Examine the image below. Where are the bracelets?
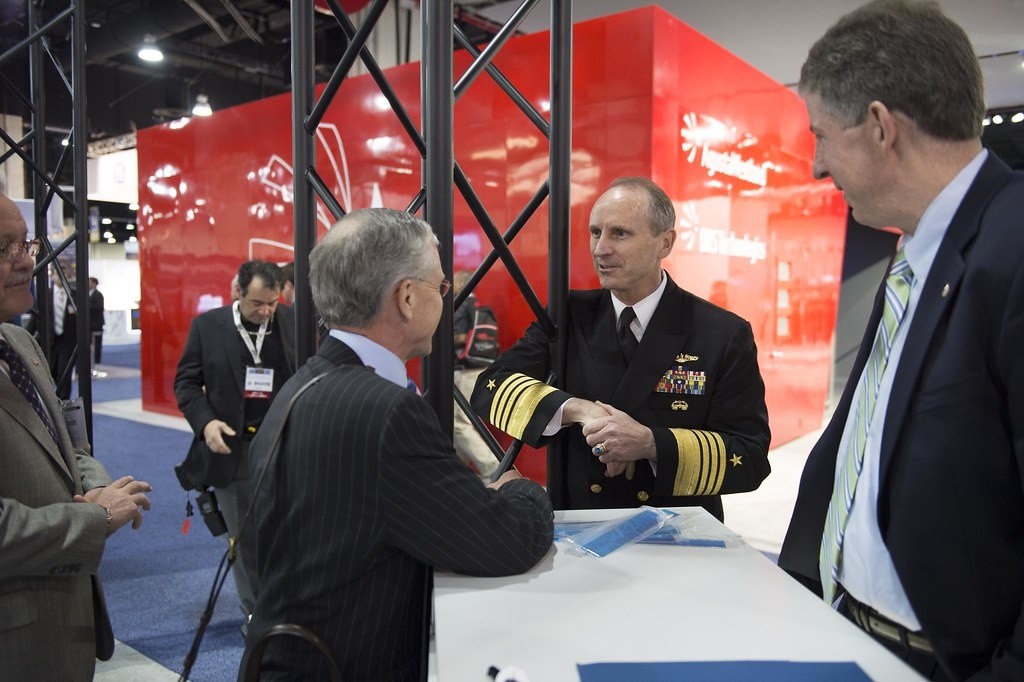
[100,504,112,527]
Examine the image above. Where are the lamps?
[191,93,213,118]
[138,32,164,63]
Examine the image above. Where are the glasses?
[394,279,451,297]
[0,239,41,260]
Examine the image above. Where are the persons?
[89,278,105,363]
[236,207,555,682]
[23,265,76,399]
[778,0,1024,682]
[468,177,772,524]
[174,260,295,621]
[453,270,500,478]
[0,194,152,682]
[280,262,296,309]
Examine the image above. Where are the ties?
[616,307,639,368]
[406,377,422,398]
[0,340,64,460]
[55,291,64,336]
[818,245,915,607]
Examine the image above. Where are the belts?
[845,601,933,654]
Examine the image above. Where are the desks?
[428,506,929,681]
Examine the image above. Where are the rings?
[595,443,607,454]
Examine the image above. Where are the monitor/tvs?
[88,200,139,244]
